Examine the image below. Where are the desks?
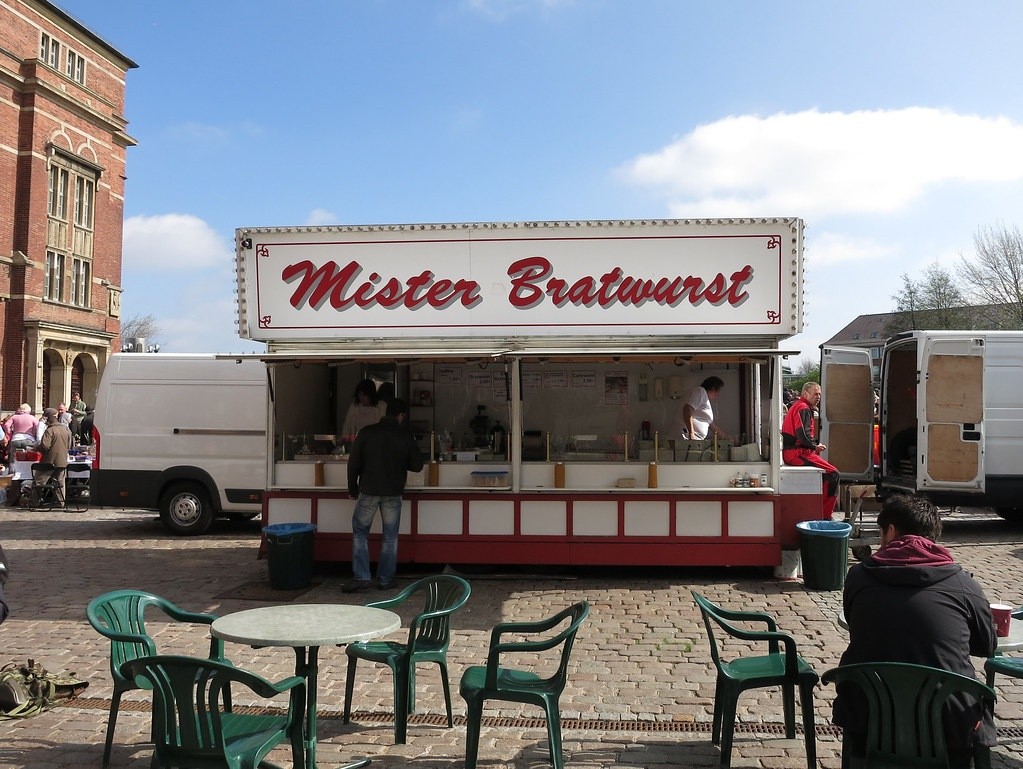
[210,603,402,769]
[838,608,1023,653]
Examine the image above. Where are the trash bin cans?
[795,520,853,592]
[262,523,318,593]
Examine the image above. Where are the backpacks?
[19,480,39,506]
[0,657,89,718]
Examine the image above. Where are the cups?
[990,604,1012,636]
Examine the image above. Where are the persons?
[0,423,8,447]
[24,416,72,510]
[81,405,95,447]
[68,392,87,426]
[339,398,424,596]
[5,403,39,468]
[58,404,72,429]
[339,380,387,452]
[833,494,999,768]
[783,381,841,520]
[376,382,394,401]
[784,386,801,417]
[39,408,59,451]
[667,376,737,448]
[69,414,82,448]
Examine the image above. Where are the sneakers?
[341,579,370,592]
[378,579,398,590]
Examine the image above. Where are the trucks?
[86,353,267,534]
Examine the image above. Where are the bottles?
[736,471,750,488]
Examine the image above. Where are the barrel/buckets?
[490,420,504,454]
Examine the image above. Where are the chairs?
[63,463,104,513]
[344,574,472,745]
[119,654,306,769]
[85,589,225,769]
[28,462,68,512]
[820,660,999,769]
[690,589,821,769]
[457,599,591,769]
[983,657,1023,720]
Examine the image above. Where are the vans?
[818,330,1022,528]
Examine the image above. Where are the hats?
[44,407,58,415]
[85,405,94,410]
